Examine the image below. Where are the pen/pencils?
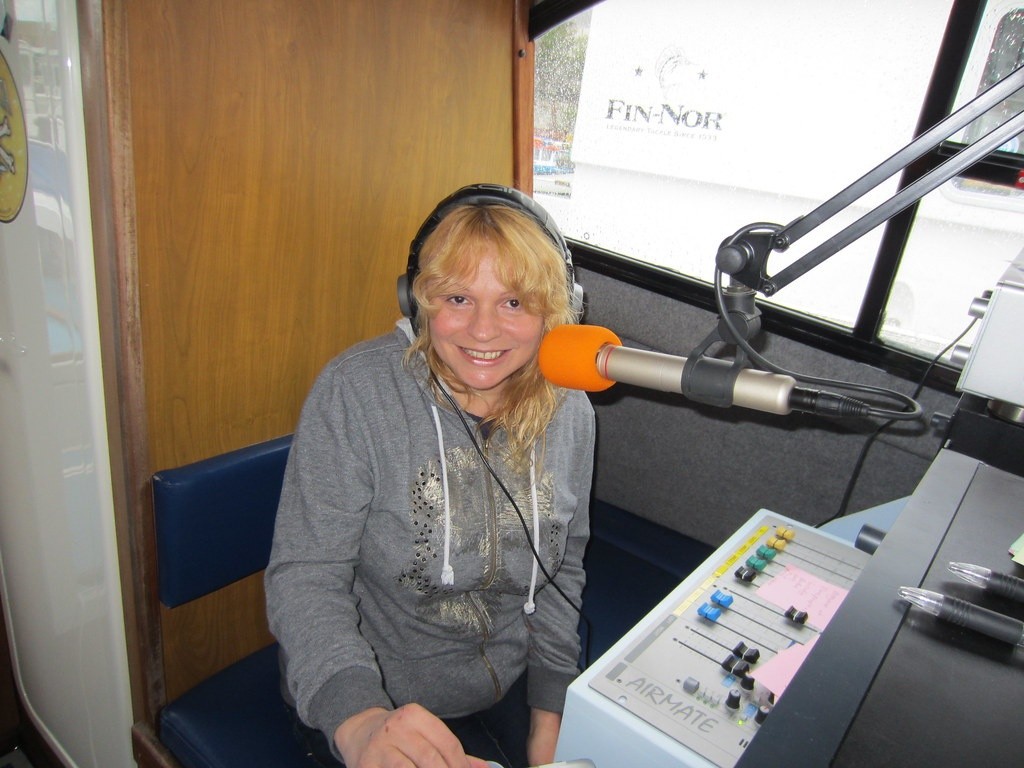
[946,560,1024,603]
[899,586,1024,649]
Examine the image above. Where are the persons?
[263,184,598,768]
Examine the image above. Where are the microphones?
[538,324,796,415]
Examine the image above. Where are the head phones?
[397,183,583,337]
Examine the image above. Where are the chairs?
[133,429,722,768]
